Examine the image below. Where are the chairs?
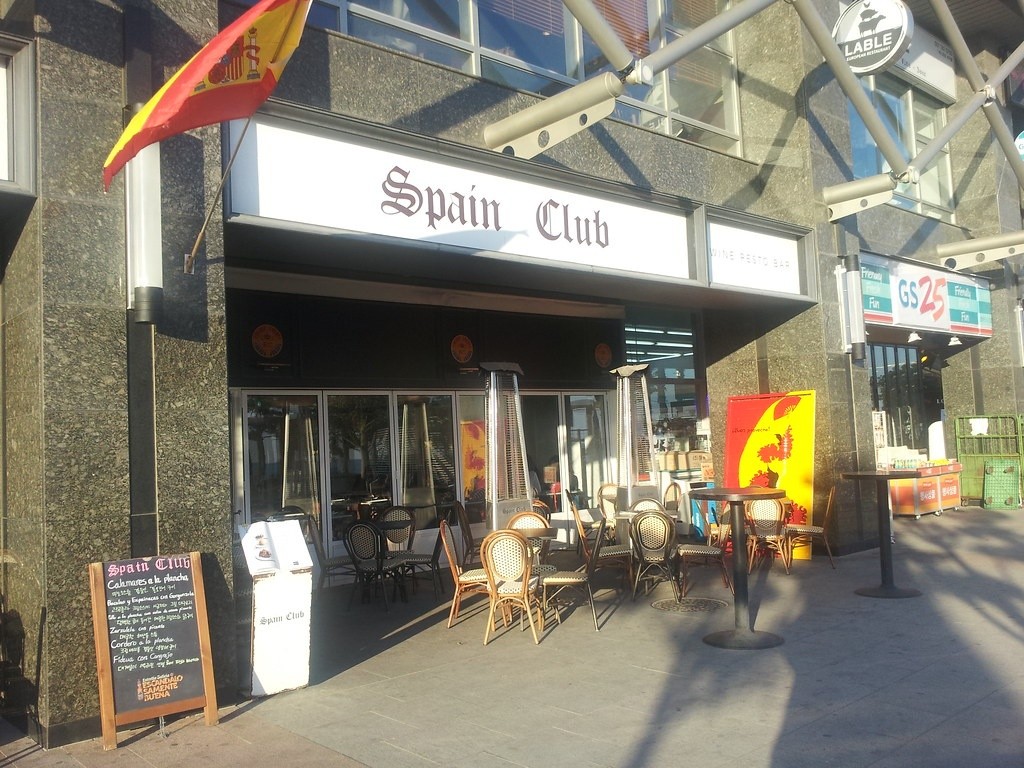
[308,483,837,646]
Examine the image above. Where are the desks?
[614,509,678,578]
[840,471,923,598]
[688,487,785,650]
[335,519,415,603]
[598,493,616,501]
[513,527,557,538]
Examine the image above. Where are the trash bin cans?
[486,498,532,535]
[615,484,661,547]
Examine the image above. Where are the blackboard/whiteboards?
[89,549,216,727]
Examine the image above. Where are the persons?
[527,454,555,512]
[548,456,580,510]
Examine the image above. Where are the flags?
[103,0,310,188]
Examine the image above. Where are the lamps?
[907,332,922,343]
[121,101,163,322]
[948,335,962,346]
[836,254,866,361]
